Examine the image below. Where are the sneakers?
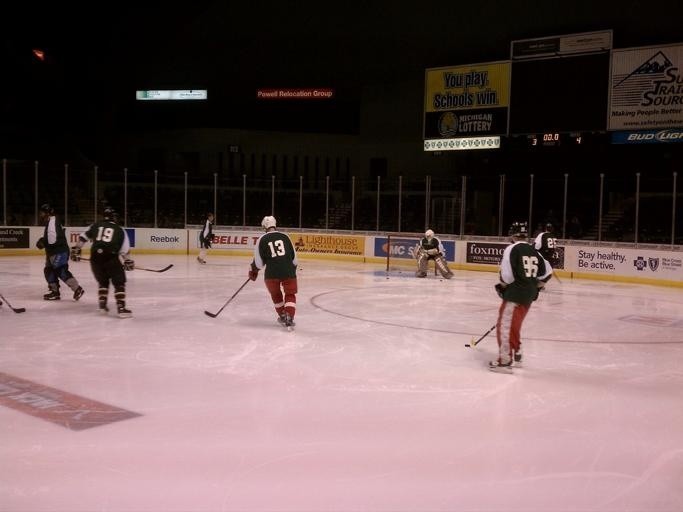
[277,314,296,327]
[118,306,132,315]
[43,292,61,298]
[487,360,513,369]
[73,286,83,299]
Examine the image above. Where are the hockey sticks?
[463,324,497,347]
[0,294,25,314]
[204,278,249,318]
[79,257,174,274]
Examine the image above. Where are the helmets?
[103,206,120,222]
[40,203,55,215]
[261,215,277,232]
[509,222,529,240]
[425,230,435,240]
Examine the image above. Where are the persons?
[533,223,558,291]
[248,216,297,332]
[414,229,456,280]
[36,203,85,302]
[487,218,554,375]
[196,212,214,265]
[71,206,135,319]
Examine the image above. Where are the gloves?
[70,246,81,261]
[123,260,135,271]
